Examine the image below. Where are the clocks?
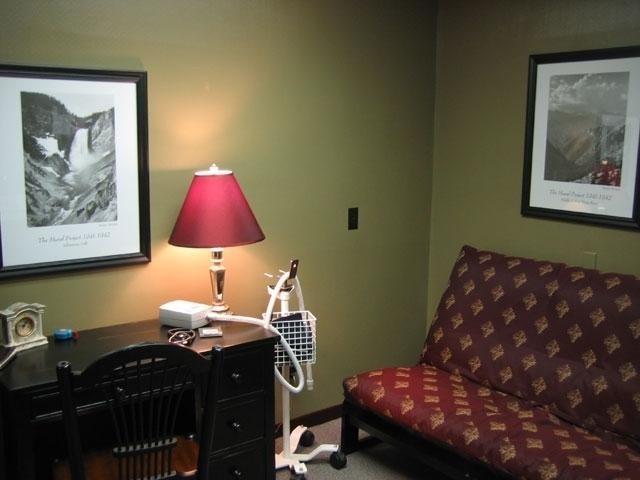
[0,302,49,352]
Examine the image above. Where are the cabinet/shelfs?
[1,310,281,479]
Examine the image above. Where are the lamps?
[168,163,264,319]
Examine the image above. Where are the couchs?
[339,247,640,480]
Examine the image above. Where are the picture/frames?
[519,47,640,234]
[0,63,150,282]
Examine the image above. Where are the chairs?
[54,341,221,480]
